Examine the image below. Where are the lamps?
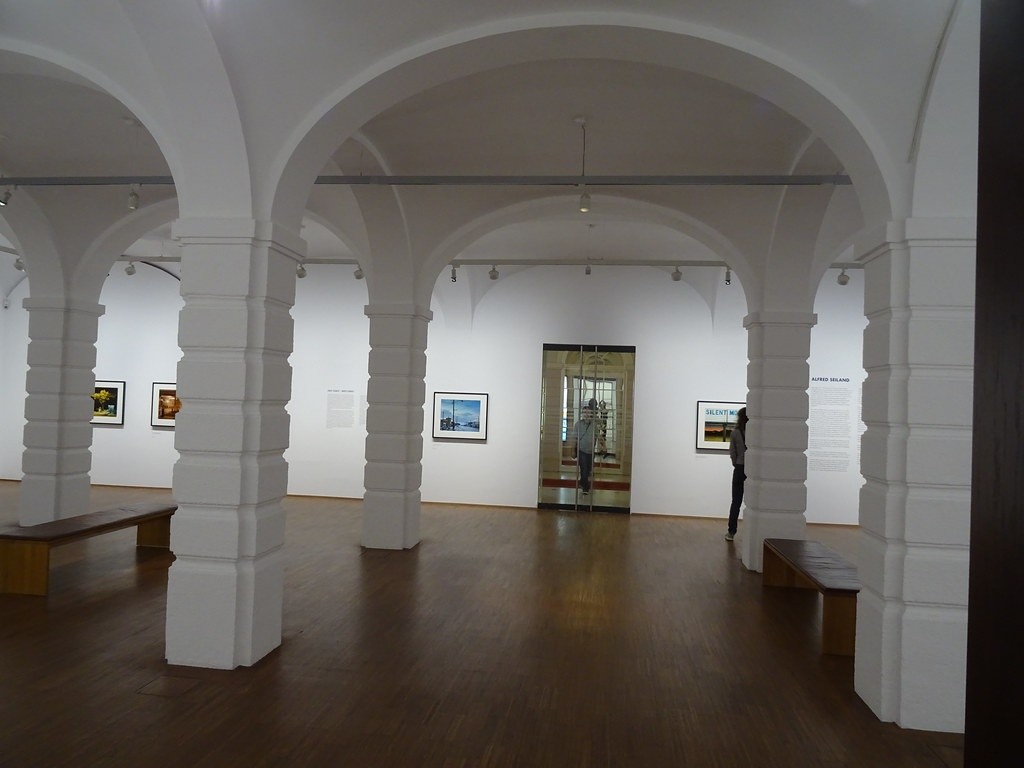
[838,268,849,284]
[354,264,364,280]
[725,267,732,285]
[13,259,26,272]
[124,262,136,274]
[296,263,306,278]
[128,192,138,209]
[488,265,499,279]
[0,188,12,206]
[451,265,457,283]
[671,267,681,281]
[574,116,592,212]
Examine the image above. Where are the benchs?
[759,537,863,661]
[0,501,179,599]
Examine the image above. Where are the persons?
[588,398,608,459]
[571,406,598,495]
[725,407,749,540]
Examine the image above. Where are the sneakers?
[724,533,733,540]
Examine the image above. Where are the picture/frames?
[432,391,488,440]
[93,380,126,425]
[696,400,745,453]
[151,381,177,428]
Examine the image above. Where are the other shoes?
[582,489,589,495]
[602,435,606,437]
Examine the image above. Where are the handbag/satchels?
[572,441,578,458]
